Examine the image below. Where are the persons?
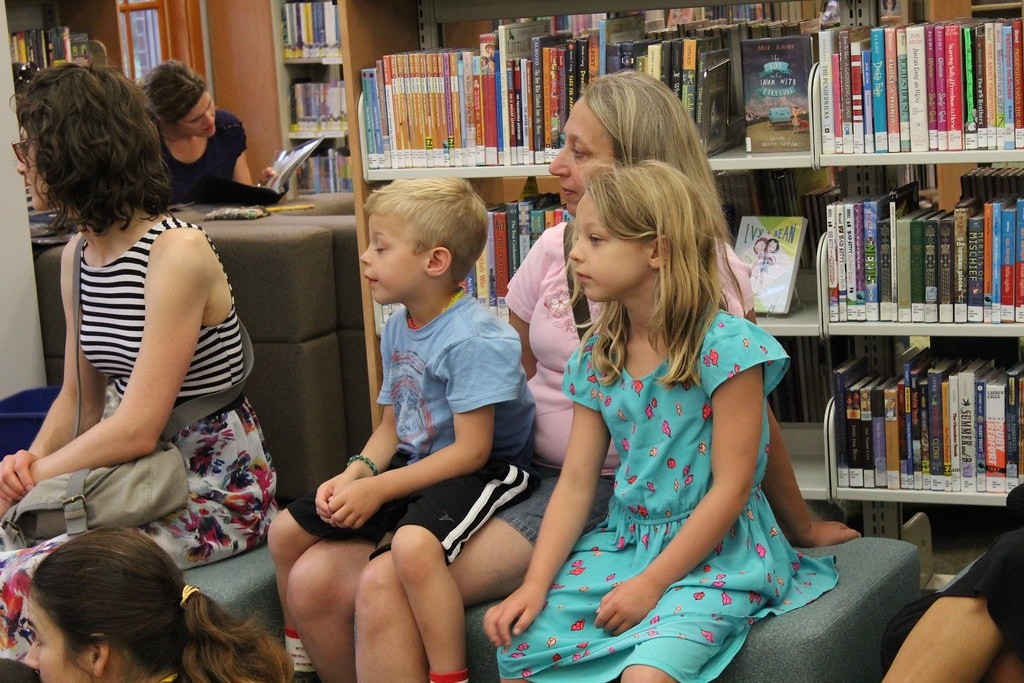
[269,72,860,683]
[0,58,294,683]
[882,484,1024,683]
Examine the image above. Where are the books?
[766,335,1024,493]
[283,0,353,195]
[365,3,1024,168]
[185,135,326,206]
[9,26,89,69]
[370,162,1024,325]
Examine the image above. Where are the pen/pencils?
[267,203,316,209]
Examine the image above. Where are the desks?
[34,190,372,497]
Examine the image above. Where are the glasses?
[11,137,38,163]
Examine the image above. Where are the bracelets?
[347,455,378,476]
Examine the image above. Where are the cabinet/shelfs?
[332,0,1024,594]
[269,0,354,195]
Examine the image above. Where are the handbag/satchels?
[2,440,189,548]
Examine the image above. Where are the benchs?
[462,538,920,683]
[183,525,282,651]
